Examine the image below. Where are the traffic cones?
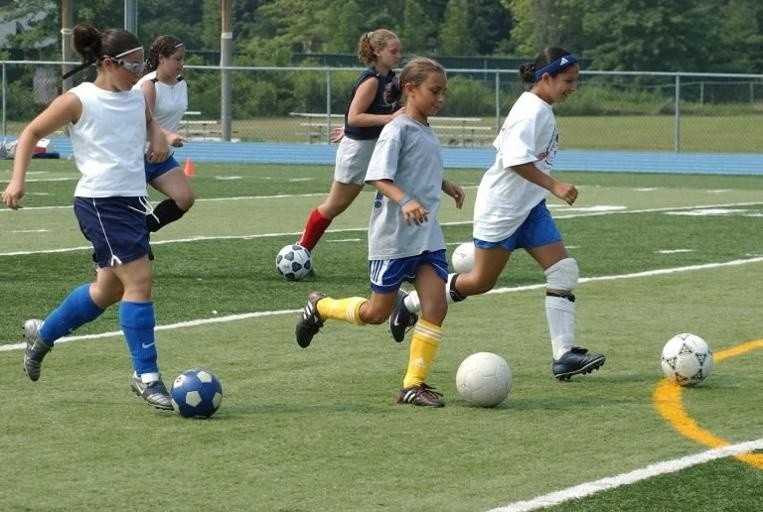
[183,158,195,176]
[184,158,193,176]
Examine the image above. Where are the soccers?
[660,332,714,387]
[455,351,513,408]
[451,242,476,275]
[276,244,313,281]
[171,367,224,421]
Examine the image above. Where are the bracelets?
[398,193,415,207]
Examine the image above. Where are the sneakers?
[295,291,327,348]
[551,346,606,381]
[22,317,54,382]
[387,288,417,342]
[129,370,173,410]
[395,382,445,407]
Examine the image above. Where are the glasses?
[102,53,146,73]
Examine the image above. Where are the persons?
[295,57,465,408]
[380,45,606,381]
[1,22,175,412]
[292,28,410,277]
[130,35,195,233]
